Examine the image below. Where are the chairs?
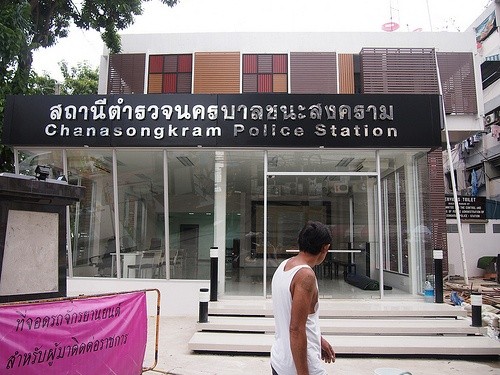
[127,249,185,279]
[89,237,115,277]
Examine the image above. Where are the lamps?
[34,165,50,180]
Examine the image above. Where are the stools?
[315,259,356,280]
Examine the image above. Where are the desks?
[110,249,141,279]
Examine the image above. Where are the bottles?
[424,281,434,303]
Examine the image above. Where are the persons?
[270,220,336,375]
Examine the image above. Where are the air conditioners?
[484,112,495,126]
